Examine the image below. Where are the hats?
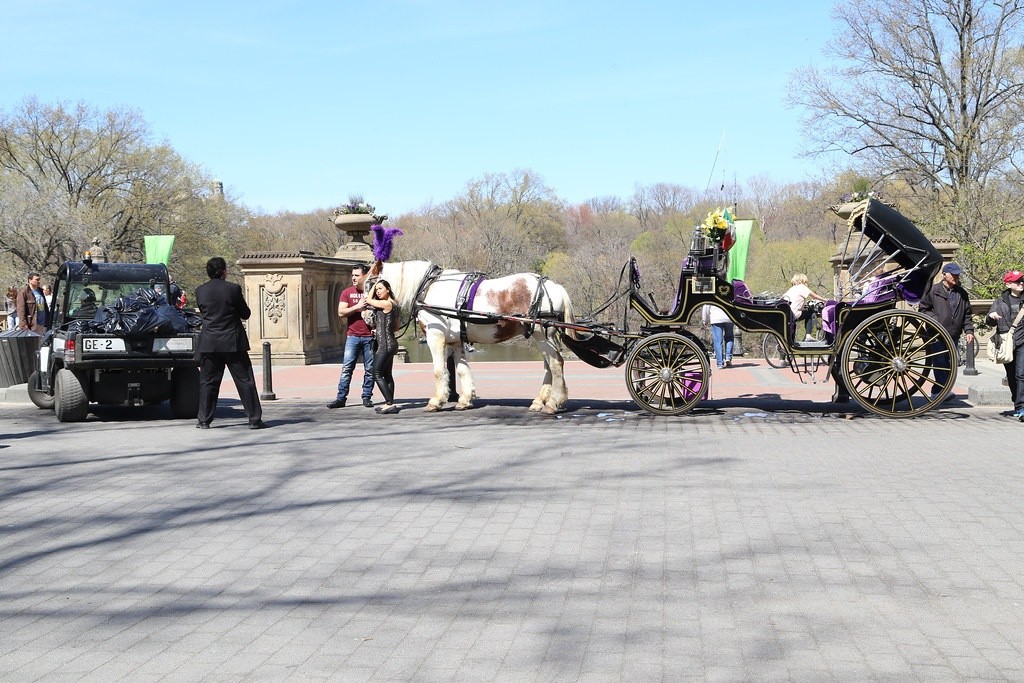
[1003,271,1024,283]
[942,263,965,274]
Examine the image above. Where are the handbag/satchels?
[986,332,1013,364]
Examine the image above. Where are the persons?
[195,257,264,429]
[327,264,375,407]
[917,263,974,402]
[4,286,20,330]
[16,273,50,340]
[702,305,734,368]
[366,279,400,414]
[73,288,97,315]
[43,284,58,313]
[986,271,1024,416]
[154,275,181,307]
[782,273,827,342]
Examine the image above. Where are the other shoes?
[718,366,723,369]
[931,390,955,402]
[375,404,398,414]
[779,361,791,367]
[725,360,732,367]
[805,337,817,342]
[326,400,345,408]
[1014,408,1024,421]
[363,399,373,407]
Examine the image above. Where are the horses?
[361,259,577,414]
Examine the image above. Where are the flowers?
[700,203,738,246]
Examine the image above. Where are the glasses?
[1015,277,1024,284]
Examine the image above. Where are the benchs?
[732,279,796,345]
[822,270,905,344]
[668,256,722,323]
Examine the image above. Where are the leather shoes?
[250,422,269,428]
[196,421,209,429]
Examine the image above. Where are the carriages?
[365,188,962,421]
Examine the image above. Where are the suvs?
[26,249,203,422]
